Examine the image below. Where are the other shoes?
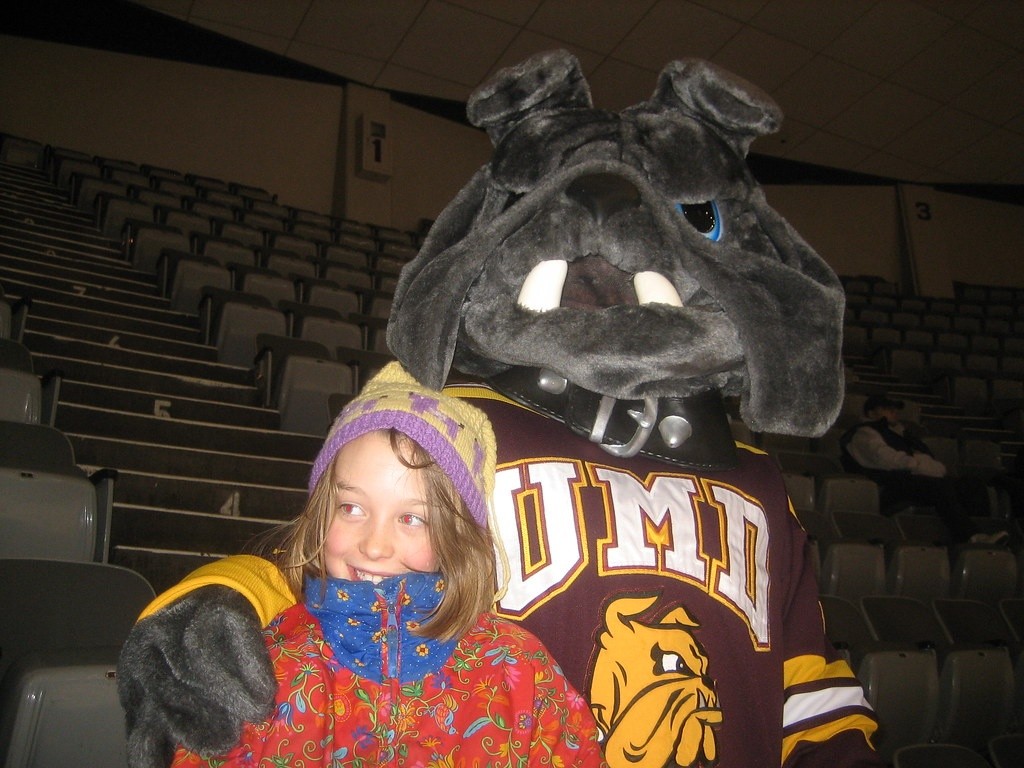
[969,531,1009,547]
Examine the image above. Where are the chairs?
[0,134,1024,768]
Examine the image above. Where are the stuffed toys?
[116,48,879,768]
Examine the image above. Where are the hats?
[863,394,904,413]
[310,362,495,531]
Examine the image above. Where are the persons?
[839,389,1009,547]
[168,361,608,768]
